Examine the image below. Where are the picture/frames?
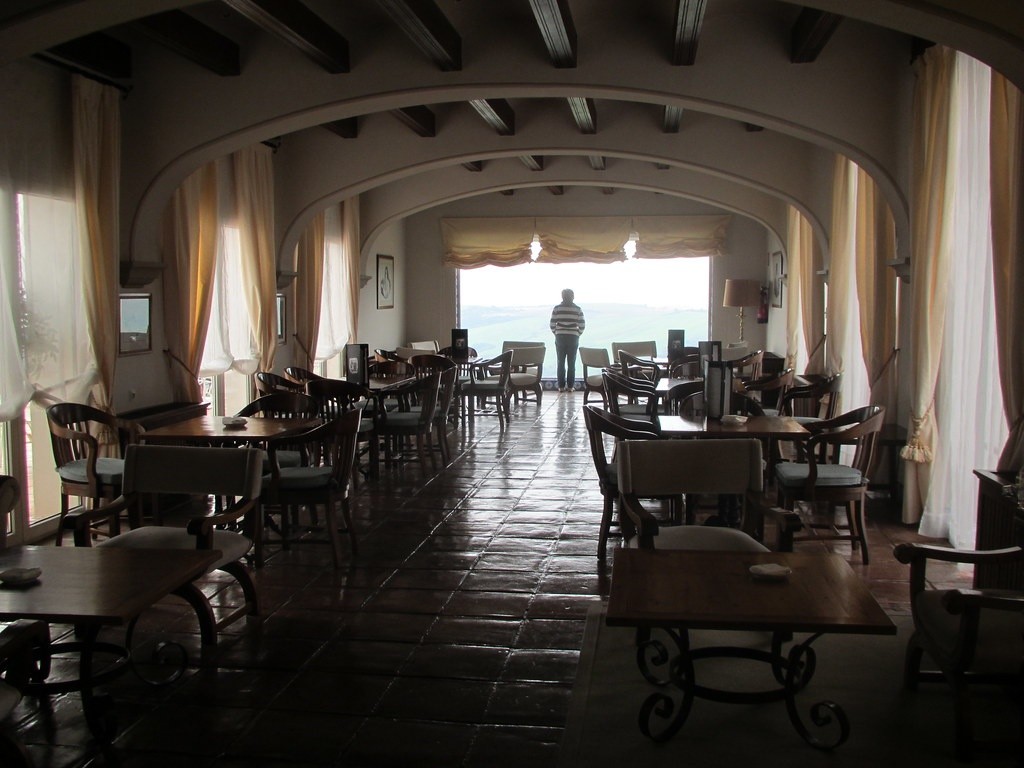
[770,251,782,308]
[376,255,394,310]
[118,293,153,357]
[276,293,287,345]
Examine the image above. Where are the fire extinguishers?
[757,286,769,320]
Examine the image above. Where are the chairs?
[0,329,1024,768]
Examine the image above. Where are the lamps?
[722,278,761,343]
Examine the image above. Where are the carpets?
[554,600,1024,768]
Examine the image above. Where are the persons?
[550,288,585,392]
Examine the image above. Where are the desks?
[607,548,897,751]
[0,543,223,749]
[655,378,746,397]
[334,376,417,481]
[447,357,483,364]
[830,423,907,504]
[656,415,811,527]
[138,416,322,560]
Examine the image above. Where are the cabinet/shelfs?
[792,374,828,417]
[118,402,210,519]
[751,350,784,404]
[972,469,1024,592]
